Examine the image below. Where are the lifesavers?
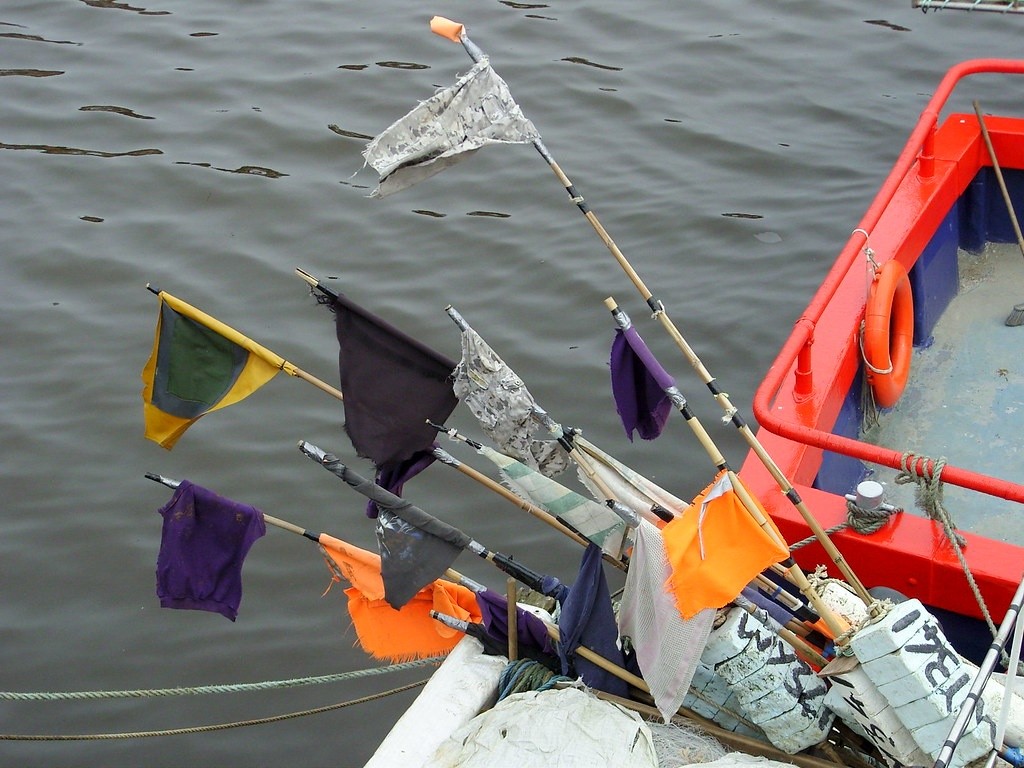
[863,260,914,407]
